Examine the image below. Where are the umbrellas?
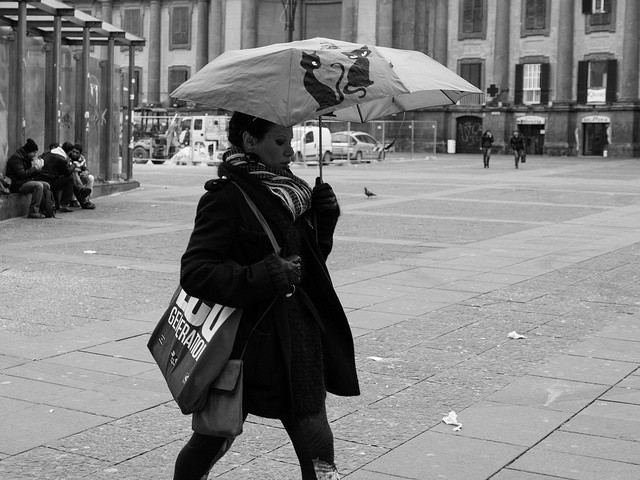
[170,37,485,181]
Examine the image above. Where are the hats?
[23,138,38,153]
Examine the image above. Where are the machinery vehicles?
[119,108,171,164]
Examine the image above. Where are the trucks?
[170,113,232,166]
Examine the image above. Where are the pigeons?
[365,187,376,198]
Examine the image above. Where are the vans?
[291,126,332,165]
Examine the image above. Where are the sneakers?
[27,212,45,219]
[76,188,91,203]
[73,200,80,207]
[58,204,73,212]
[82,201,95,209]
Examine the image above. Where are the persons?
[509,131,525,168]
[184,125,190,145]
[69,144,94,207]
[49,142,91,208]
[41,142,74,212]
[480,130,494,168]
[173,112,361,479]
[6,138,51,218]
[179,125,186,143]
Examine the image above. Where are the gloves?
[313,173,339,222]
[257,249,303,288]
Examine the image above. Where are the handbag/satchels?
[145,285,243,415]
[191,360,243,438]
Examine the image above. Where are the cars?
[331,131,385,162]
